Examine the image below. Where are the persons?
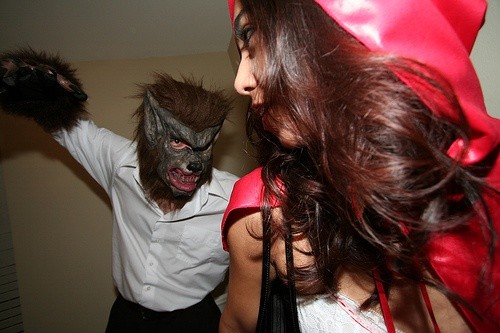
[221,0,500,333]
[0,47,242,333]
